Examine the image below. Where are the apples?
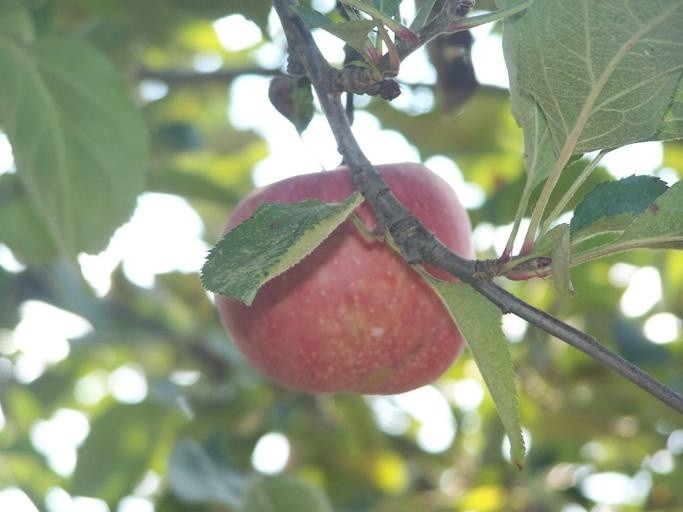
[215,162,474,394]
